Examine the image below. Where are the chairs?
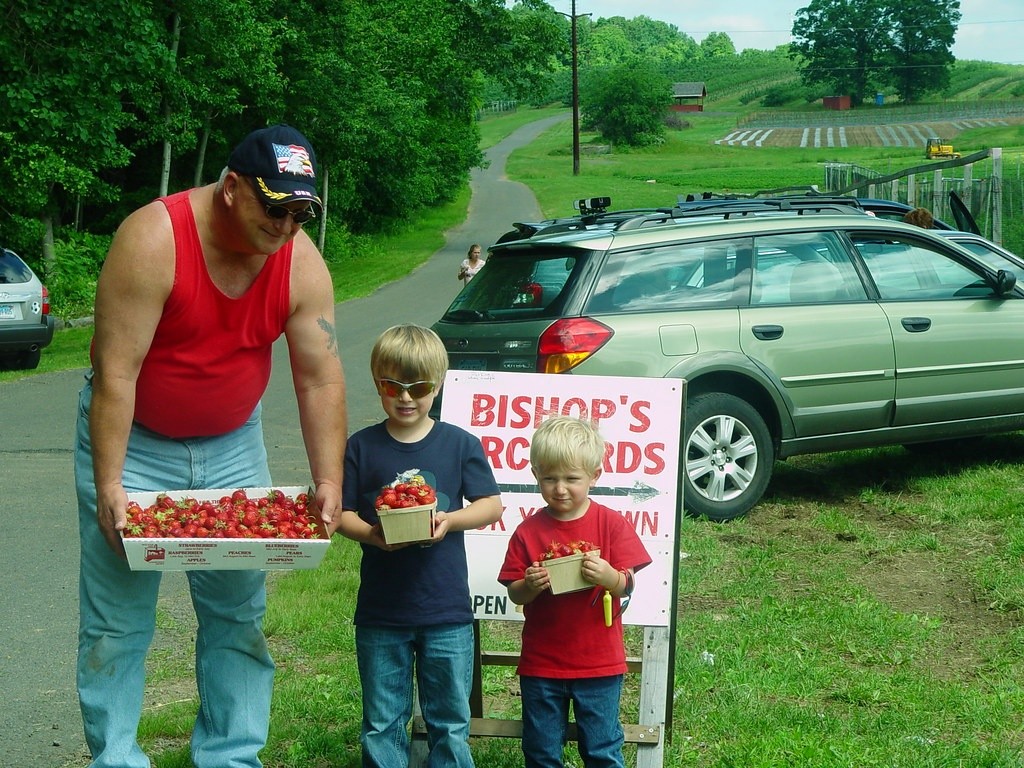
[704,267,763,304]
[789,260,849,302]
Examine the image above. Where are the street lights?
[553,0,593,177]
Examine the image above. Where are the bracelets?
[603,572,621,626]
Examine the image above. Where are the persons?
[497,413,653,768]
[904,207,935,230]
[74,125,348,768]
[335,322,503,768]
[458,244,485,287]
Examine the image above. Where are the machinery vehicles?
[926,136,962,161]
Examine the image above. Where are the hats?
[228,123,323,214]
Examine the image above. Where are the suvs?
[431,190,1024,522]
[1,248,56,371]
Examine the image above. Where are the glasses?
[374,378,436,399]
[244,176,316,224]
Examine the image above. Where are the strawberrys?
[538,539,594,562]
[121,488,321,539]
[375,479,437,511]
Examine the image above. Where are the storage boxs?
[542,550,601,594]
[376,498,437,545]
